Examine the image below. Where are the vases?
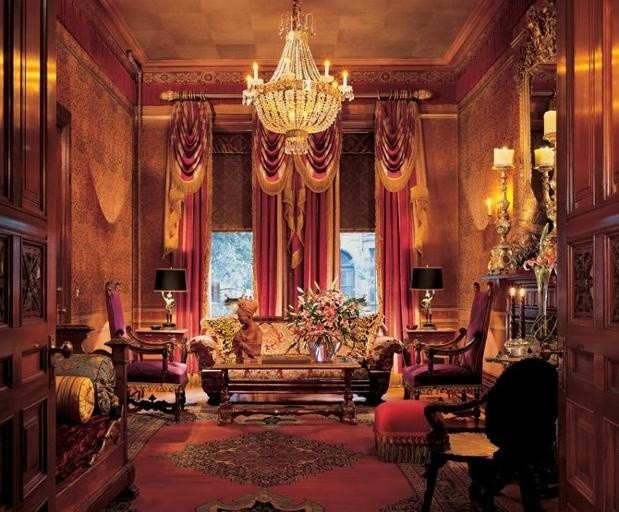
[308,341,340,362]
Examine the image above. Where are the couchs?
[186,311,404,406]
[54,321,139,512]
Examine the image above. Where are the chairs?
[402,281,493,423]
[104,280,189,423]
[422,357,559,512]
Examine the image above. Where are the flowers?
[283,278,367,358]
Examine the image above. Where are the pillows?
[53,352,119,417]
[55,374,95,424]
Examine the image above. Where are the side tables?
[403,328,457,344]
[135,326,187,361]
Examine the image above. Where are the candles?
[507,288,516,340]
[543,110,556,134]
[534,146,554,165]
[493,146,514,164]
[519,289,527,338]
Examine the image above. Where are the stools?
[374,400,429,464]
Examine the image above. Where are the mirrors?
[514,4,557,212]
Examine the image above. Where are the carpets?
[107,400,523,512]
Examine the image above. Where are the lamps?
[410,267,444,328]
[241,0,355,156]
[153,267,187,328]
